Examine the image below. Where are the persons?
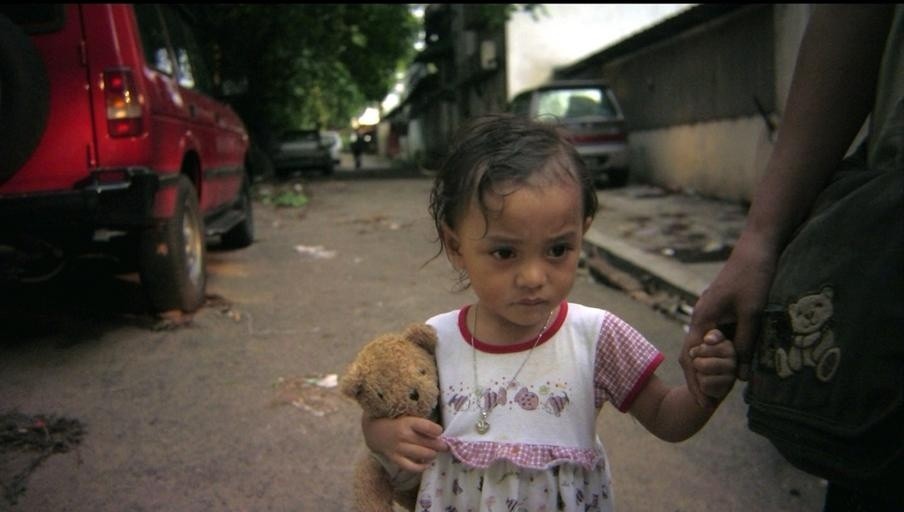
[360,108,738,512]
[678,3,904,512]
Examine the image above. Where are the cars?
[270,125,344,181]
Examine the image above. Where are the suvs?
[0,0,257,317]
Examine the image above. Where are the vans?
[504,76,630,189]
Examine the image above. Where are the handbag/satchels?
[740,147,903,501]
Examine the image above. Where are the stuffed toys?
[337,321,440,512]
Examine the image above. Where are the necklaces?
[472,299,554,434]
